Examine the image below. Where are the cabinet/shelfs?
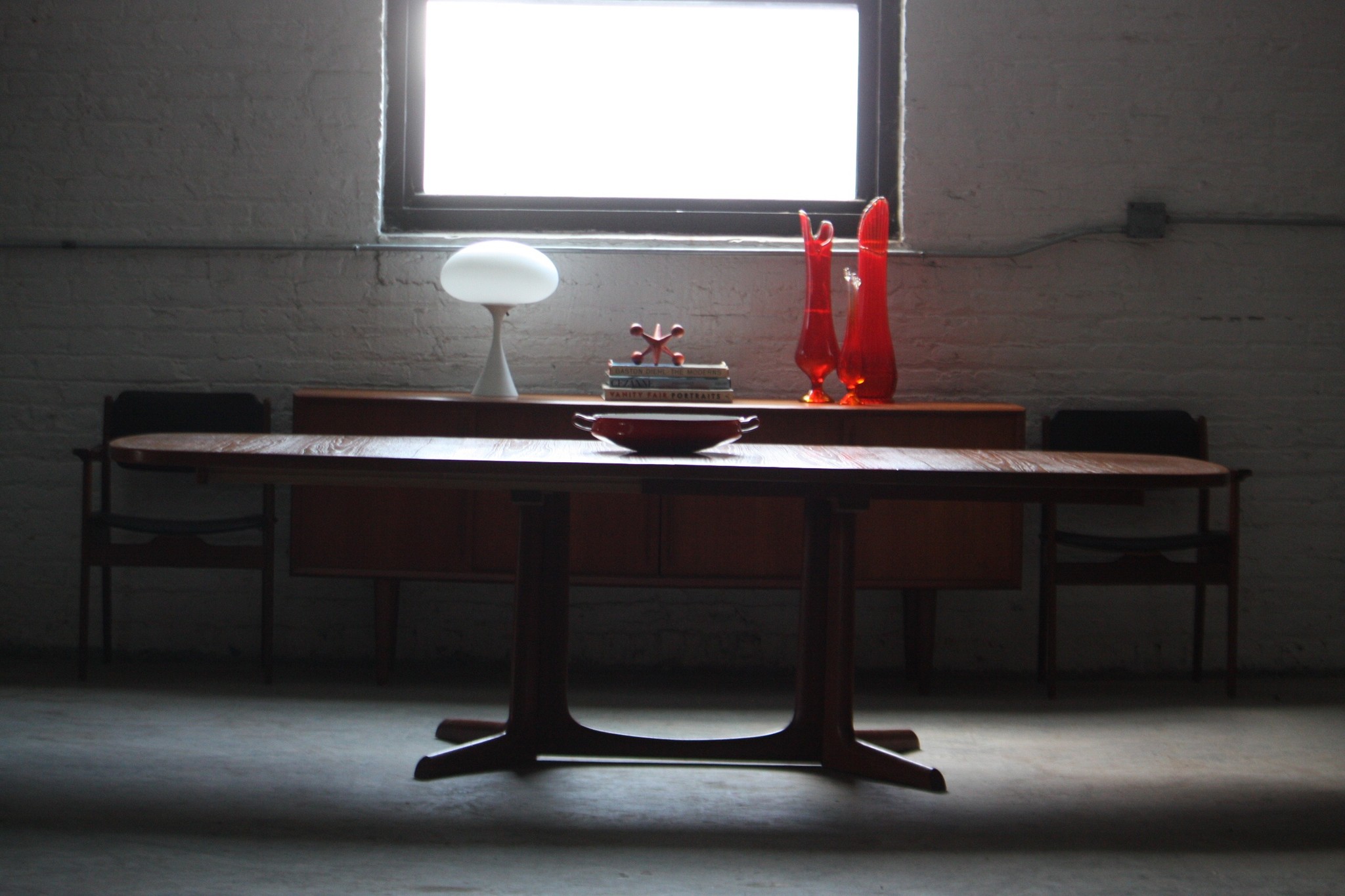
[289,391,1029,690]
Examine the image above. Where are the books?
[602,362,734,403]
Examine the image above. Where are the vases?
[839,196,903,404]
[794,209,839,406]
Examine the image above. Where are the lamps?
[440,241,559,401]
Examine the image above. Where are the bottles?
[838,196,900,406]
[793,209,841,403]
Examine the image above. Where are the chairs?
[1026,409,1242,689]
[75,387,285,675]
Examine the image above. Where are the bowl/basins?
[572,410,761,456]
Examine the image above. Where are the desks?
[111,427,1232,789]
